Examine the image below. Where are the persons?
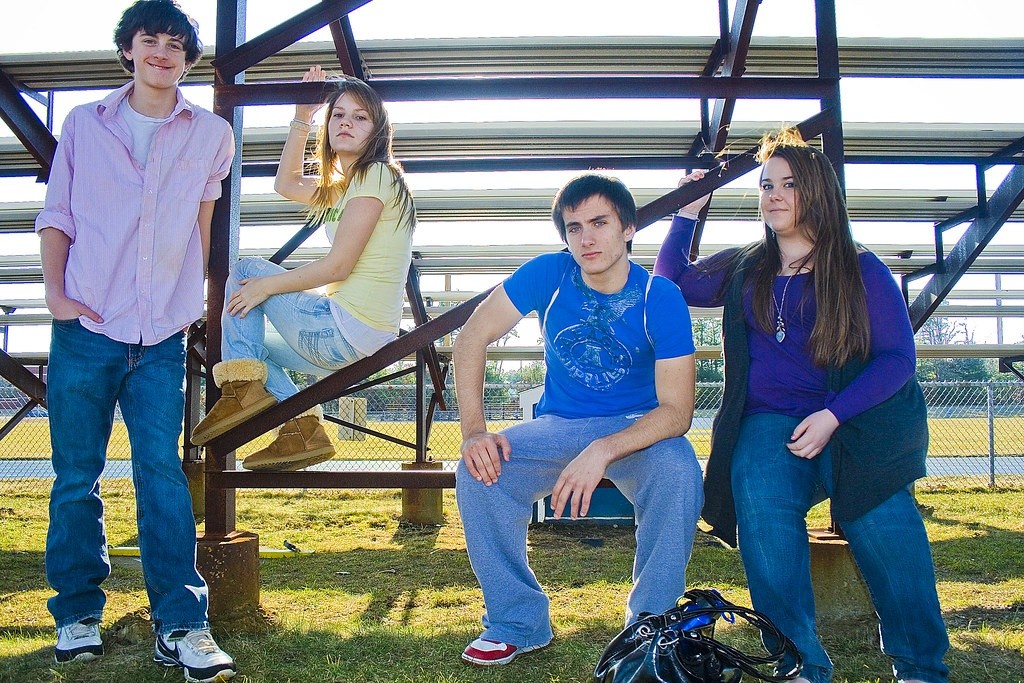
[36,0,238,683]
[653,141,954,683]
[452,173,705,666]
[190,64,419,471]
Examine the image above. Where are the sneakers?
[54,617,104,665]
[461,636,554,666]
[152,627,237,683]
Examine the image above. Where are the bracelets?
[290,119,312,132]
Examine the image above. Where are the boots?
[242,403,337,471]
[190,359,277,447]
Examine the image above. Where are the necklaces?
[772,269,799,343]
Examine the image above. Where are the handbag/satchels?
[593,588,805,683]
